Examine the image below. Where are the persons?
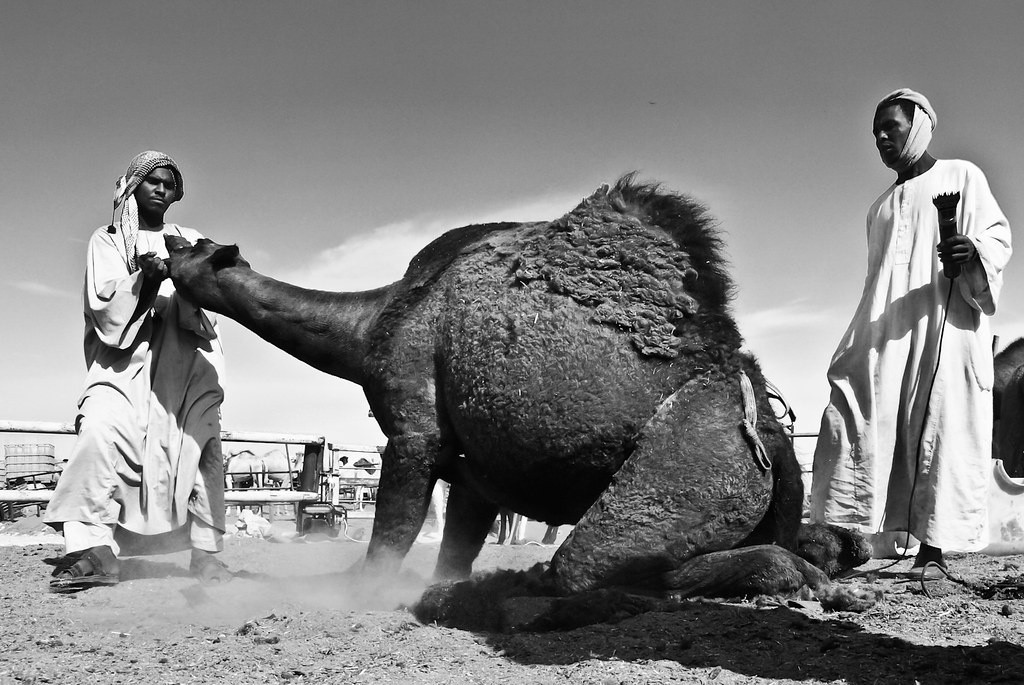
[810,88,1023,560]
[42,149,242,595]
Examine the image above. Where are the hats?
[108,150,184,235]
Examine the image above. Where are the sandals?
[50,551,127,588]
[188,553,230,588]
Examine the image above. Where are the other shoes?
[908,549,944,579]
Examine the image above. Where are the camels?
[162,173,875,634]
